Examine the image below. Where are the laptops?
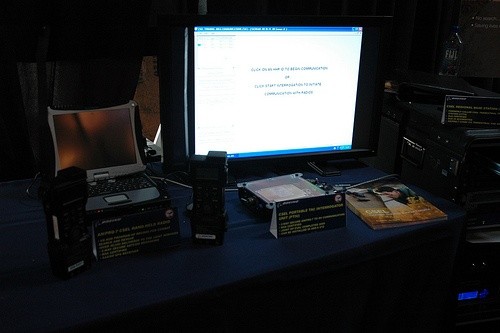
[47,99,160,212]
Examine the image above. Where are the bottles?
[438,25,464,77]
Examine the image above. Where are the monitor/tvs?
[185,14,394,185]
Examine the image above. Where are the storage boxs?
[236,172,329,223]
[90,189,182,261]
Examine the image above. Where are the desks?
[0,163,466,333]
